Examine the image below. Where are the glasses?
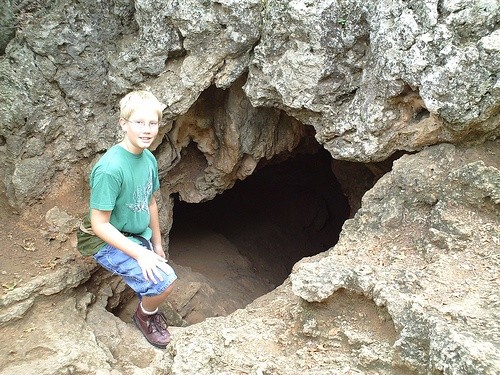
[123,116,161,128]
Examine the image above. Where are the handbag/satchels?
[76,212,152,258]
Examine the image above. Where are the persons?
[90,91,177,348]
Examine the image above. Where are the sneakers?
[132,301,172,347]
